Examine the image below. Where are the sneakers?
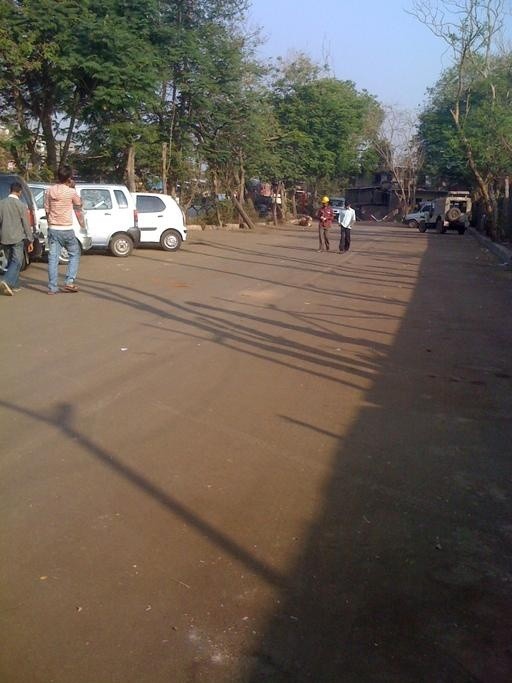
[64,284,80,292]
[0,280,21,296]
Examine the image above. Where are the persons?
[317,196,356,254]
[0,182,35,296]
[43,165,81,295]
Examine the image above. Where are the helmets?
[321,196,330,203]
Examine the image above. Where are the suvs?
[399,201,435,231]
[416,190,474,237]
[327,195,347,220]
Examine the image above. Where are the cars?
[0,183,187,274]
